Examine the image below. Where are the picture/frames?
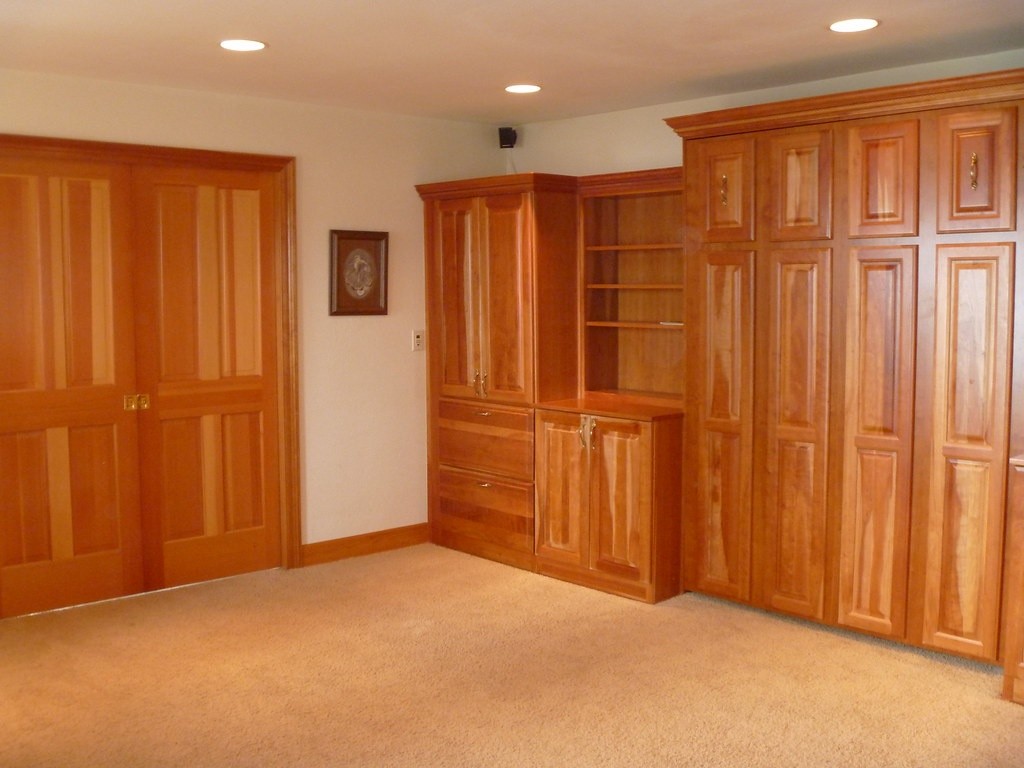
[327,229,390,317]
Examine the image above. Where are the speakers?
[499,127,514,149]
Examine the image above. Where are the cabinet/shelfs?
[578,165,684,405]
[429,463,534,575]
[413,173,578,404]
[532,397,683,605]
[660,66,1023,708]
[427,395,537,483]
